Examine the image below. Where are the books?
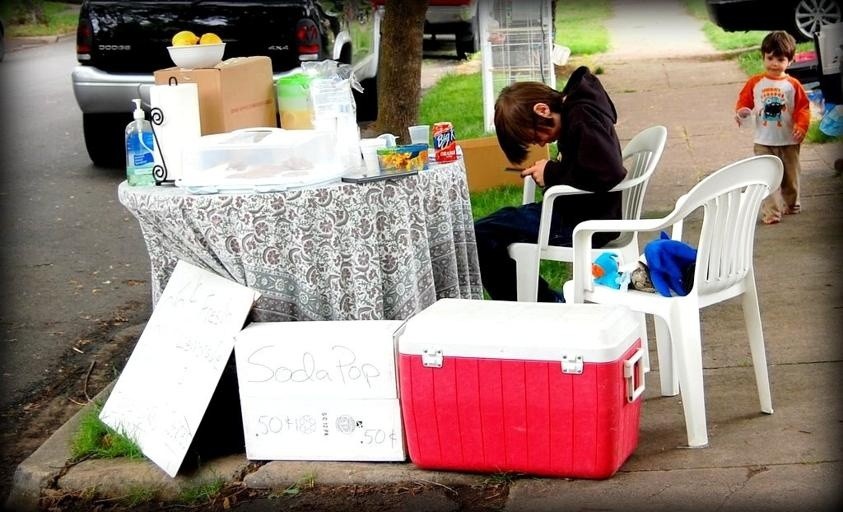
[340,168,418,185]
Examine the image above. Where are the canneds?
[432,122,457,163]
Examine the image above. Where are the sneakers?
[766,214,780,224]
[788,204,801,214]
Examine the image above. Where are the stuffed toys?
[590,251,624,289]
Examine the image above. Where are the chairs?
[508,124,668,373]
[561,156,783,449]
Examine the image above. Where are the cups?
[360,138,386,172]
[408,125,430,144]
[736,107,756,135]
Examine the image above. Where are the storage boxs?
[153,55,278,136]
[397,296,644,482]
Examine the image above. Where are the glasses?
[531,129,542,145]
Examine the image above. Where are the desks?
[117,143,483,322]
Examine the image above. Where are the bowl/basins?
[164,43,226,69]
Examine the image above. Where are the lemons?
[171,30,223,46]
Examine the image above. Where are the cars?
[705,2,841,44]
[70,2,381,166]
[420,4,480,63]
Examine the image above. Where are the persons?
[735,29,811,226]
[471,66,628,303]
